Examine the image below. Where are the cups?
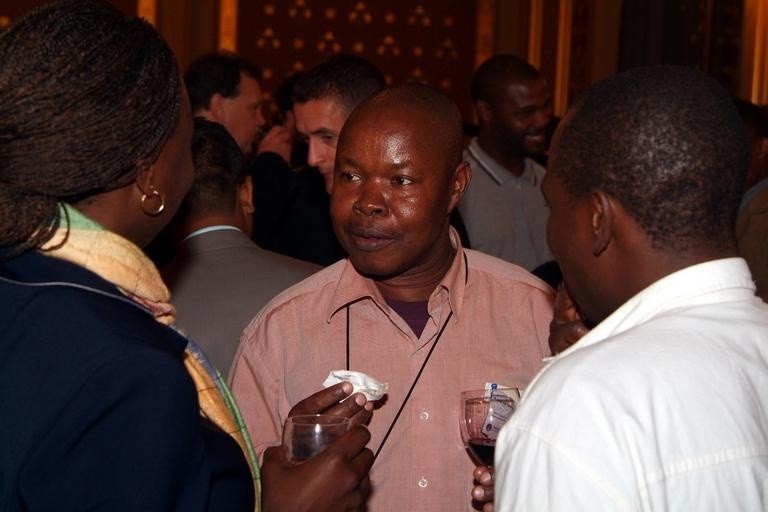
[280,413,347,461]
[458,388,520,467]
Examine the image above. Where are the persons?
[150,48,767,383]
[0,0,375,511]
[493,58,767,511]
[221,83,556,510]
